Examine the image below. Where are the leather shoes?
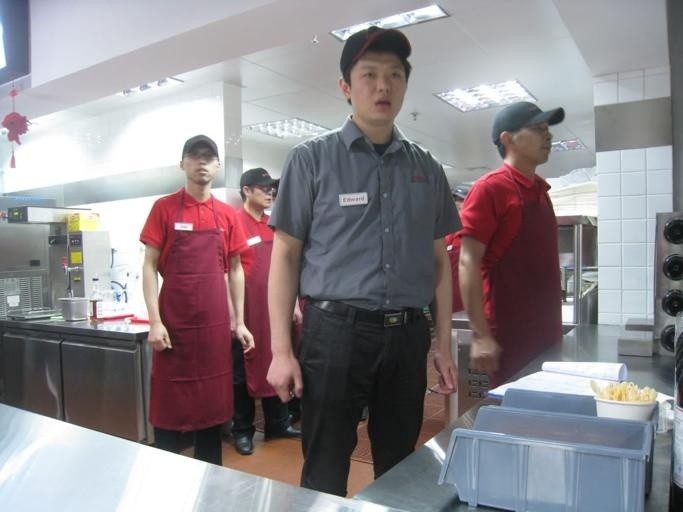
[288,410,300,423]
[235,425,255,455]
[265,425,302,441]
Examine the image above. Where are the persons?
[456,100,565,391]
[139,135,255,465]
[265,26,463,498]
[233,168,302,456]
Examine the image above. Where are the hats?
[453,186,468,199]
[183,136,218,158]
[240,167,280,187]
[340,26,411,77]
[492,101,564,143]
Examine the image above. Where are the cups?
[593,391,656,425]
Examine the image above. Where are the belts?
[310,300,424,327]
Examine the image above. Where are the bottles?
[668,331,682,512]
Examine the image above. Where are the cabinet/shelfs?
[3,328,146,442]
